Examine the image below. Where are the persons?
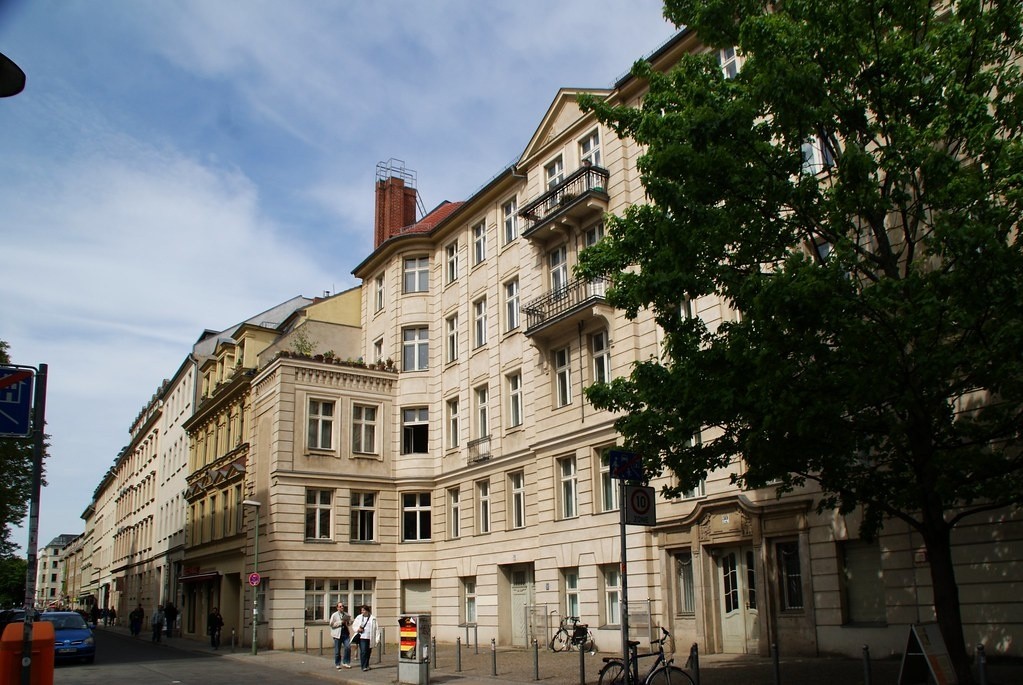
[101,606,109,628]
[90,603,98,626]
[108,606,116,627]
[328,602,352,670]
[129,608,137,637]
[150,604,164,647]
[133,603,144,639]
[208,607,222,650]
[351,605,380,672]
[163,602,178,638]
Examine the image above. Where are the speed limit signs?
[624,485,656,526]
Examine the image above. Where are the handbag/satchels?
[349,633,360,645]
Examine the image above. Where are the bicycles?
[597,626,696,685]
[548,617,593,653]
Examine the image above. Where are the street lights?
[242,499,261,656]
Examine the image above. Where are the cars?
[0,610,35,639]
[39,612,95,664]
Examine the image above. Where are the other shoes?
[362,667,371,671]
[336,663,351,669]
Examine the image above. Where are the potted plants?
[193,322,400,404]
